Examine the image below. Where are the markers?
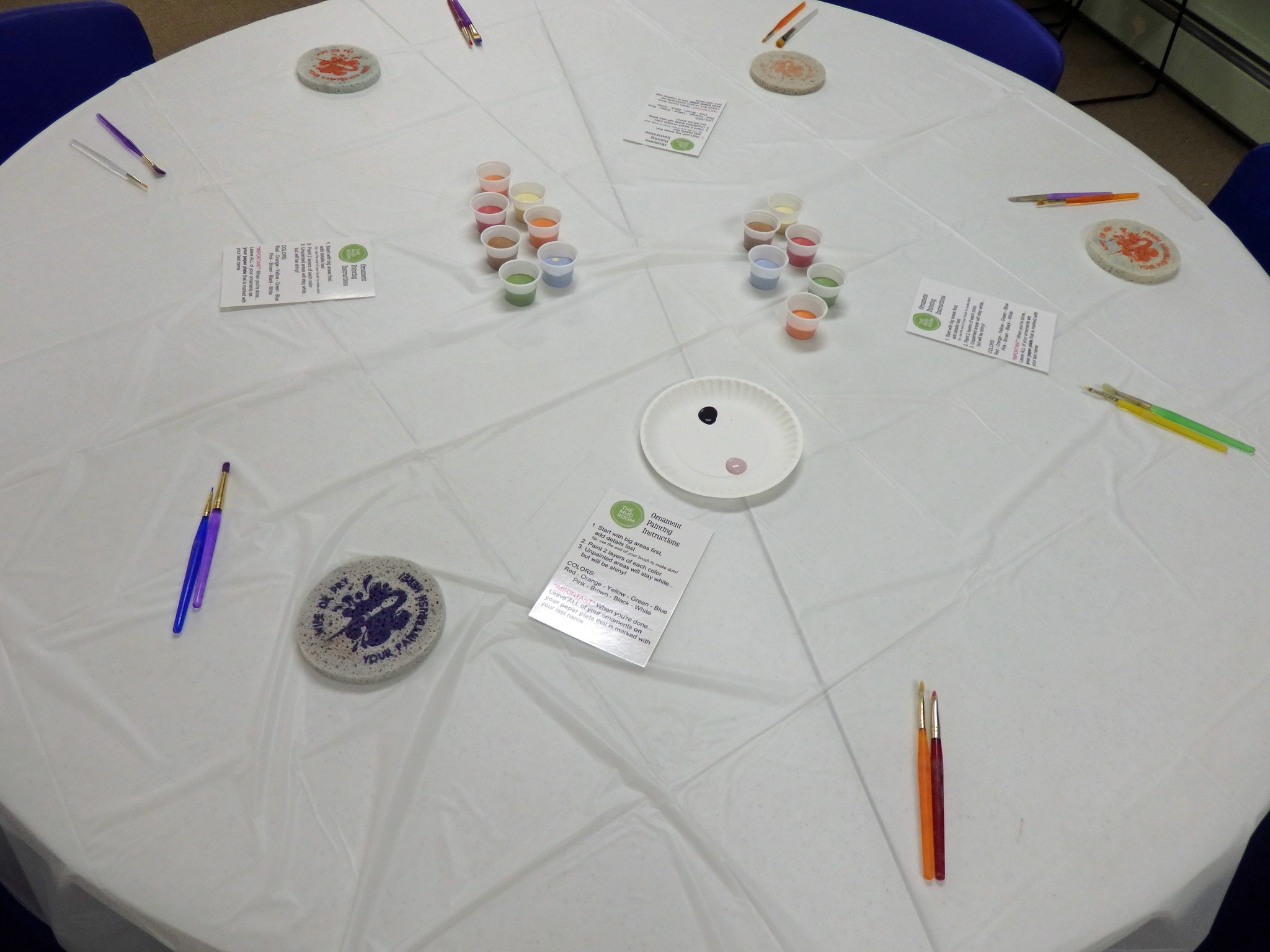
[1008,192,1114,203]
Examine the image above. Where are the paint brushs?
[69,138,148,189]
[447,0,473,45]
[775,8,819,48]
[917,681,934,879]
[761,1,808,43]
[1082,384,1229,453]
[1036,192,1140,207]
[95,112,166,175]
[453,0,483,42]
[172,488,216,635]
[1102,384,1255,454]
[193,460,231,610]
[930,690,946,880]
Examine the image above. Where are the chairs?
[0,2,158,175]
[817,1,1064,93]
[1201,143,1270,283]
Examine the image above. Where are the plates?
[640,373,804,500]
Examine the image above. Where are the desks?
[0,2,1270,949]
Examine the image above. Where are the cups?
[471,159,579,306]
[739,187,847,345]
